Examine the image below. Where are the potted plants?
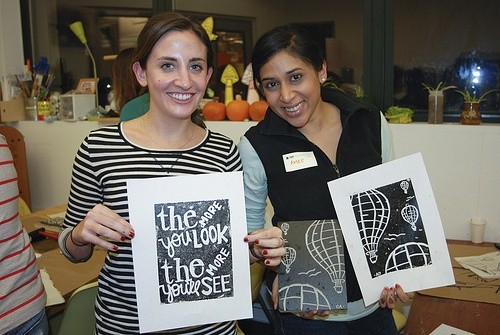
[353,86,376,105]
[385,106,414,124]
[454,88,500,125]
[421,82,457,123]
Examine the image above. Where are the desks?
[402,239,500,335]
[20,202,109,335]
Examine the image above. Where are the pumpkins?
[226,95,250,121]
[249,99,269,121]
[202,98,226,121]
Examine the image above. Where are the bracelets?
[71,222,89,246]
[249,245,263,260]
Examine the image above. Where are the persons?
[58,12,286,335]
[114,47,152,122]
[238,26,409,335]
[0,134,49,335]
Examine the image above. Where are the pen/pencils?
[14,73,23,90]
[45,73,55,91]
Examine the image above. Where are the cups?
[36,101,51,120]
[471,218,487,244]
[24,97,38,120]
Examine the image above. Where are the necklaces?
[141,116,195,173]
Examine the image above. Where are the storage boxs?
[59,94,95,119]
[0,93,25,123]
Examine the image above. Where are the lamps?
[70,21,105,120]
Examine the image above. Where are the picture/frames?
[77,77,101,93]
